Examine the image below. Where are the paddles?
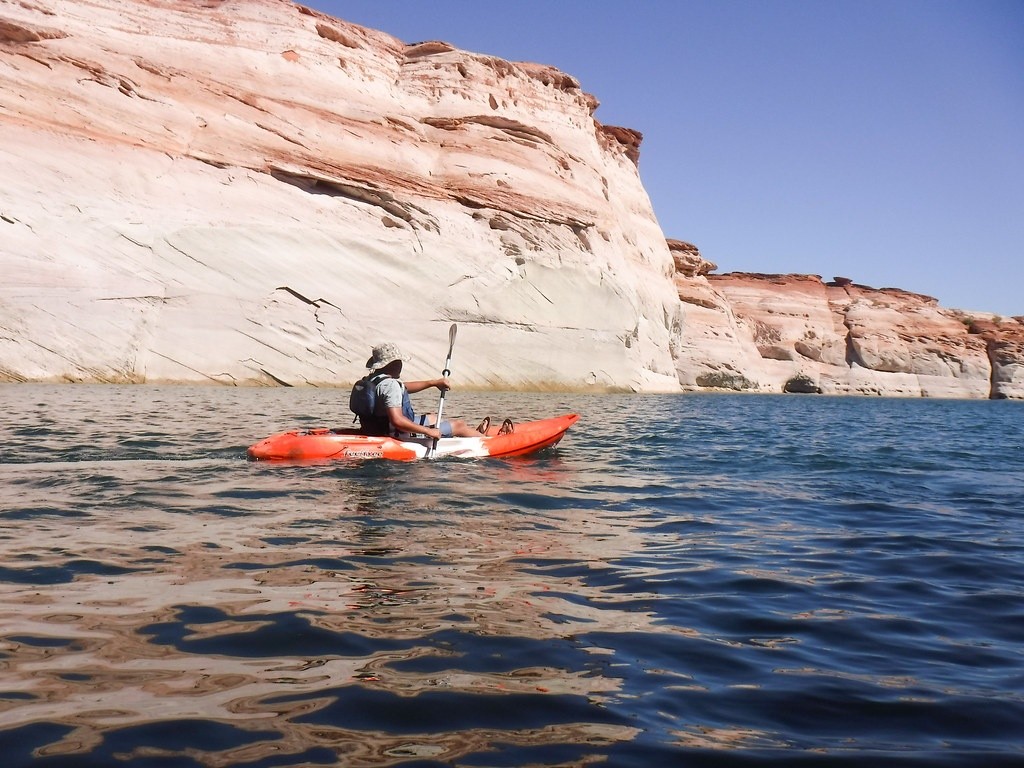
[429,323,457,462]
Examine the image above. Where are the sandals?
[497,418,514,434]
[475,416,492,436]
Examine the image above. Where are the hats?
[365,342,413,370]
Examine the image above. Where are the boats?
[247,412,581,461]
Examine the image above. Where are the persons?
[360,342,514,441]
